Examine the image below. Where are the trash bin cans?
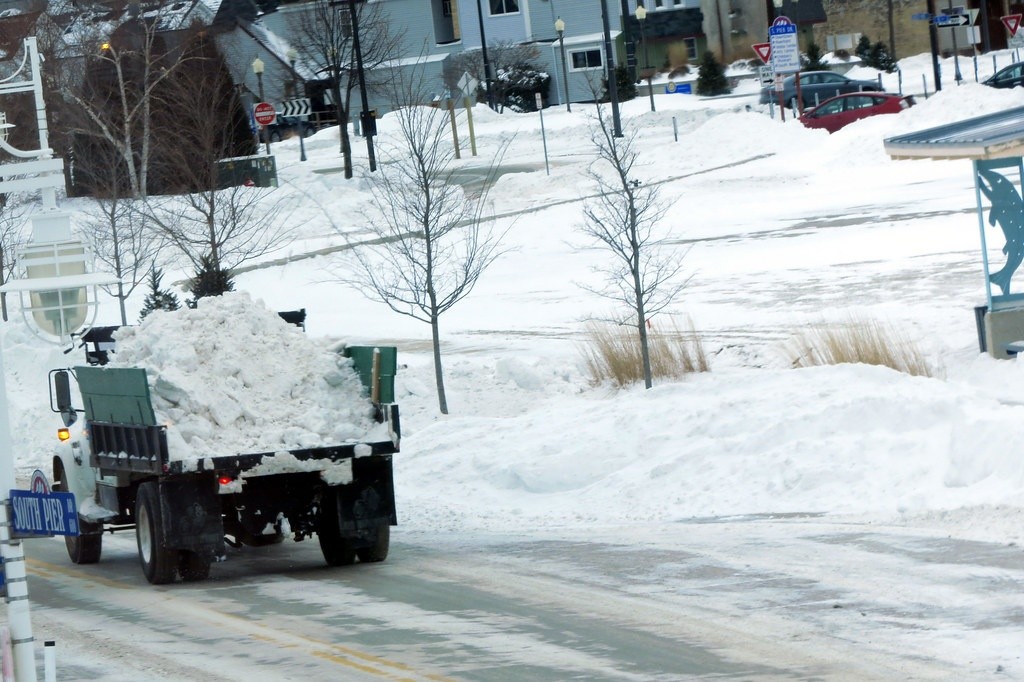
[215,155,278,188]
[360,109,377,136]
[975,306,988,353]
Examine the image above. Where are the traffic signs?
[937,14,970,28]
[759,64,775,85]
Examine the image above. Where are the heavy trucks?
[47,309,404,585]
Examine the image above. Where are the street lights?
[252,57,271,154]
[554,17,572,113]
[286,47,307,161]
[635,6,657,111]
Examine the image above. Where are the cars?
[798,92,917,135]
[981,61,1024,89]
[760,70,885,110]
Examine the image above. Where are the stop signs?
[254,102,275,125]
